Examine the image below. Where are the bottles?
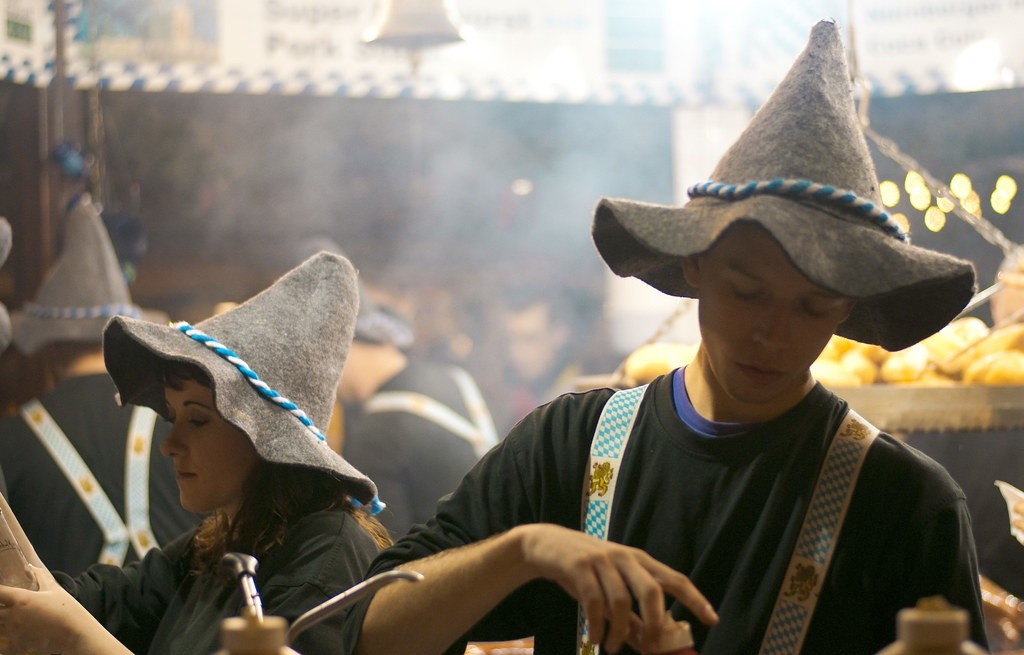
[875,607,989,655]
[210,615,301,655]
[642,612,698,655]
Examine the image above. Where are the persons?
[0,144,594,655]
[341,23,987,655]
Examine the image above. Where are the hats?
[9,200,170,353]
[591,20,977,351]
[102,252,386,515]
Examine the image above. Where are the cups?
[0,501,39,608]
